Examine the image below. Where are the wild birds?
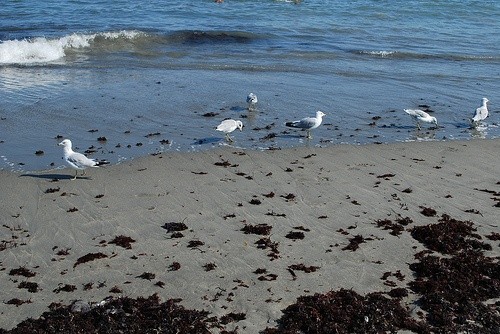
[286,111,326,138]
[403,108,438,130]
[215,117,242,141]
[472,98,489,123]
[246,92,257,108]
[58,139,110,180]
